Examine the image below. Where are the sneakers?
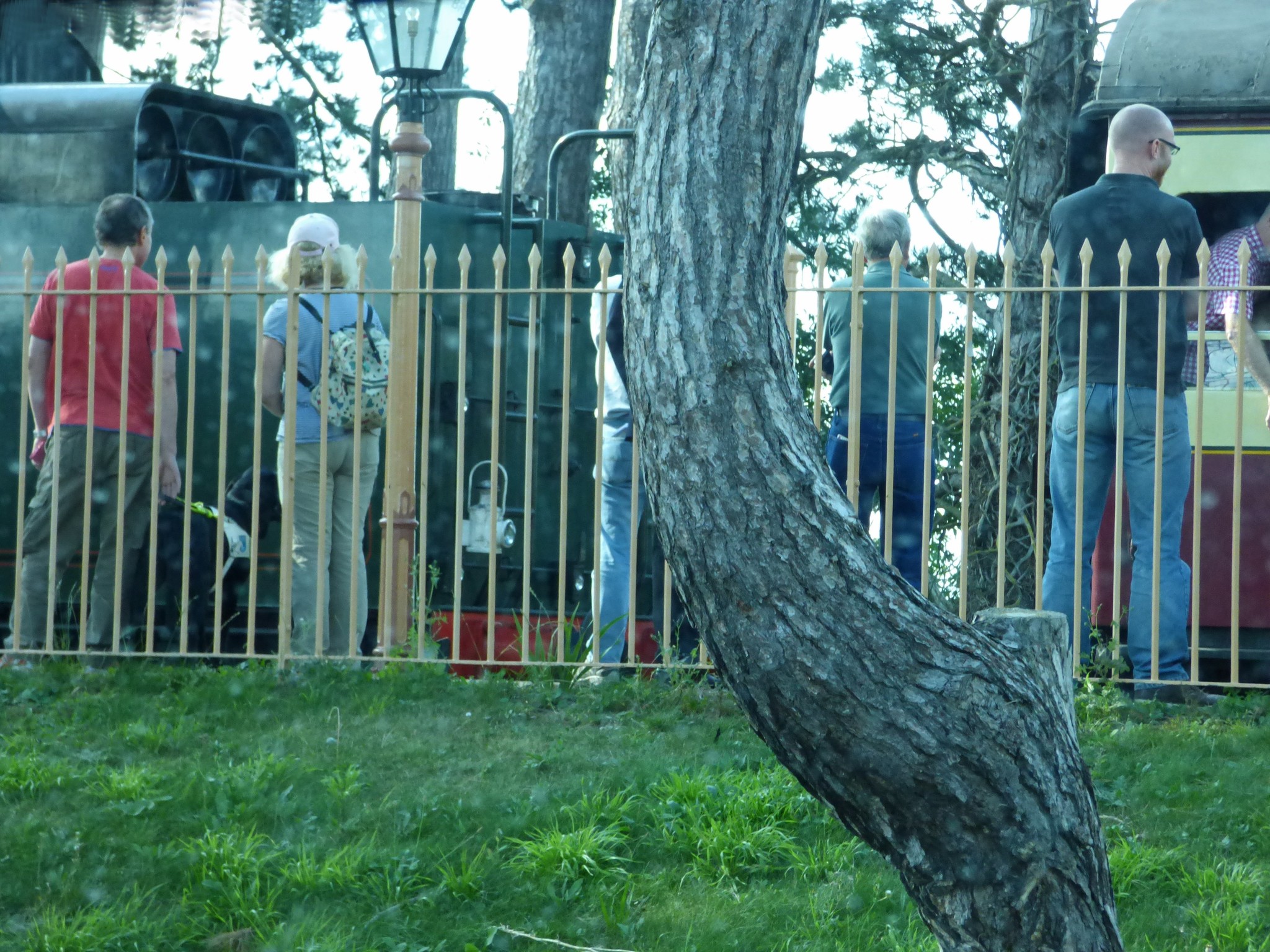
[569,657,620,688]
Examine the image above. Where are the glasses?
[1149,138,1180,156]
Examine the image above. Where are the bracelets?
[32,429,47,437]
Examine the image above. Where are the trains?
[1078,0,1270,683]
[0,76,703,676]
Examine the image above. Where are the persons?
[3,194,183,658]
[1041,103,1226,711]
[808,210,942,594]
[253,213,386,667]
[1182,202,1270,430]
[572,274,723,686]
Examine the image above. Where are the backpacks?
[295,296,391,430]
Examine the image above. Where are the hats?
[287,212,340,256]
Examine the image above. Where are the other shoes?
[0,647,38,669]
[81,647,125,677]
[1133,678,1228,709]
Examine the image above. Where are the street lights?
[340,0,475,667]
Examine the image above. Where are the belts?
[836,407,925,423]
